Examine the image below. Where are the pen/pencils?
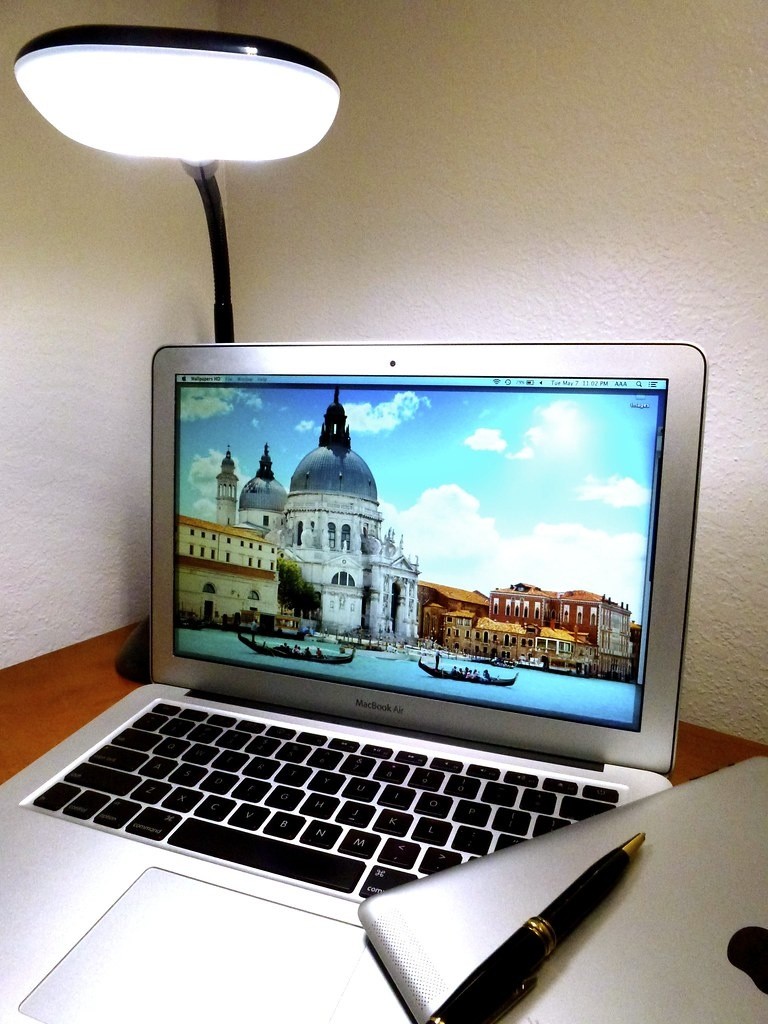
[425,831,646,1024]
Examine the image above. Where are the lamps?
[13,23,343,684]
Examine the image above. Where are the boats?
[179,602,204,630]
[416,652,519,686]
[490,657,518,669]
[237,632,353,663]
[342,644,443,663]
[220,607,306,640]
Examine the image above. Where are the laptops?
[0,343,707,1024]
[358,755,768,1024]
